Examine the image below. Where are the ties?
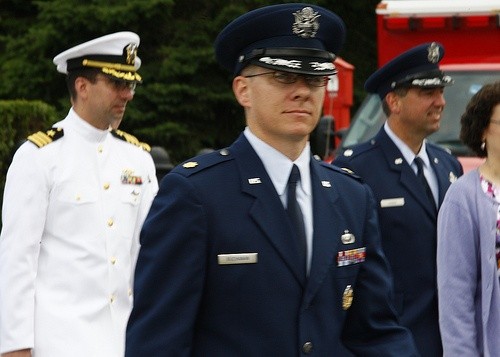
[413,157,438,216]
[285,164,307,279]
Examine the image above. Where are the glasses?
[244,71,332,87]
[88,78,136,92]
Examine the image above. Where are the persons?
[332,41,465,357]
[436,83,500,357]
[0,30,159,357]
[124,4,417,357]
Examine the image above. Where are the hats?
[214,4,346,76]
[364,42,455,101]
[53,31,144,83]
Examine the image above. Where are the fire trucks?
[308,0,500,180]
